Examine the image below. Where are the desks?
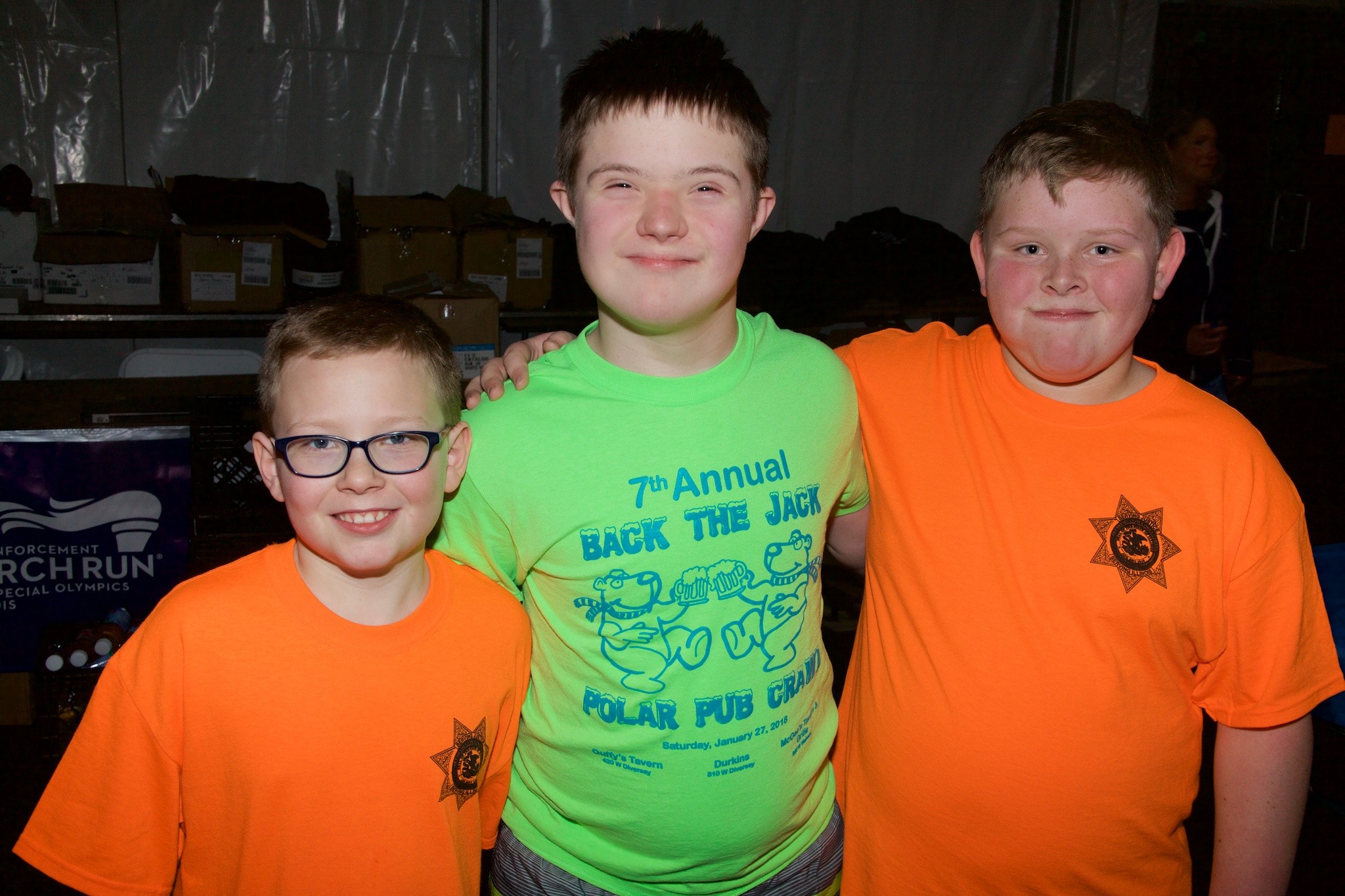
[0,378,290,730]
[0,269,602,342]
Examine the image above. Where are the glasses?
[275,428,451,479]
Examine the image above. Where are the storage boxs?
[0,163,590,384]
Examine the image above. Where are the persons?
[12,295,533,896]
[465,100,1345,896]
[415,22,871,896]
[1132,90,1254,404]
